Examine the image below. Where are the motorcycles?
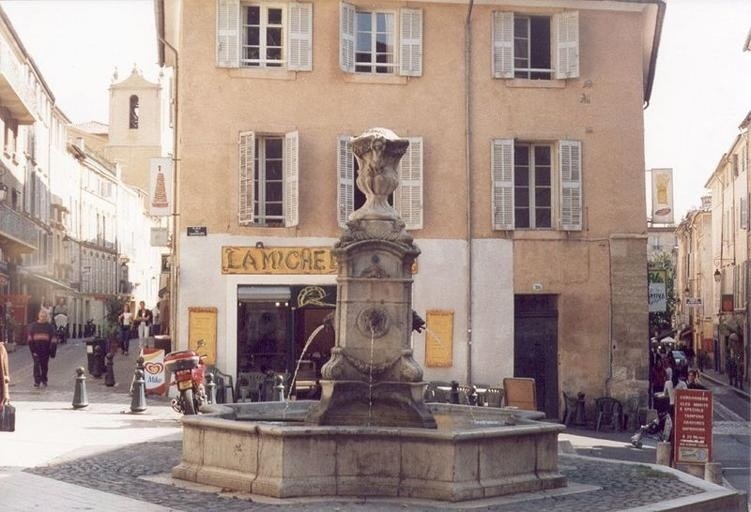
[163,338,208,415]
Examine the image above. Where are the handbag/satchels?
[0,403,15,431]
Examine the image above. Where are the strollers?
[631,404,673,448]
[53,312,68,344]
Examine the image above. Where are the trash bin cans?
[296,360,316,400]
[86,338,106,376]
[155,335,171,356]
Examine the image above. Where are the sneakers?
[33,383,40,388]
[42,382,49,386]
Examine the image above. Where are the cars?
[672,350,688,375]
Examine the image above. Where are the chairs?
[561,391,641,434]
[203,366,293,404]
[425,380,504,407]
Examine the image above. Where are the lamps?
[256,241,264,248]
[684,278,701,296]
[712,258,736,284]
[61,229,69,248]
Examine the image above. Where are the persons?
[649,340,712,439]
[25,309,58,388]
[36,296,70,344]
[370,136,388,175]
[240,311,335,401]
[115,300,160,355]
